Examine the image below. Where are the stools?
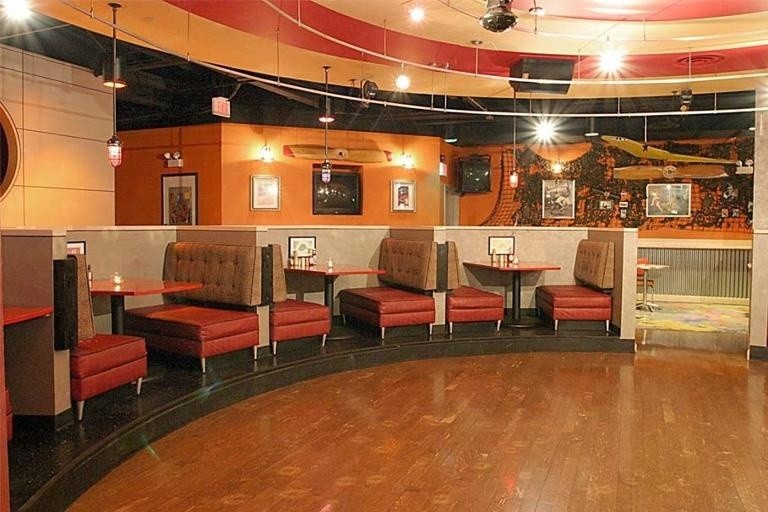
[637,262,660,314]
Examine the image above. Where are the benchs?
[44,255,149,422]
[340,234,438,344]
[443,239,505,336]
[126,239,261,377]
[533,239,616,332]
[261,245,332,354]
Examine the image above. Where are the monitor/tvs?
[313,171,361,215]
[455,153,493,193]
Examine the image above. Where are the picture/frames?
[250,175,281,213]
[160,171,199,226]
[642,182,692,220]
[389,178,417,213]
[541,178,576,220]
[310,160,365,216]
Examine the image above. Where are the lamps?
[314,94,337,125]
[317,64,335,186]
[92,45,128,91]
[163,150,184,169]
[102,2,125,170]
[503,55,579,97]
[505,82,520,191]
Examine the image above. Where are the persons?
[550,196,568,214]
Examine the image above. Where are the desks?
[284,262,387,341]
[462,255,561,329]
[2,303,53,329]
[91,273,202,335]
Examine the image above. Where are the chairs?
[637,258,656,303]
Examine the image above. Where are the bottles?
[290,249,318,266]
[88,280,93,289]
[86,264,93,281]
[112,272,122,284]
[492,248,519,264]
[327,257,334,267]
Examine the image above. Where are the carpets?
[634,296,754,337]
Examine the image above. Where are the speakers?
[509,57,575,94]
